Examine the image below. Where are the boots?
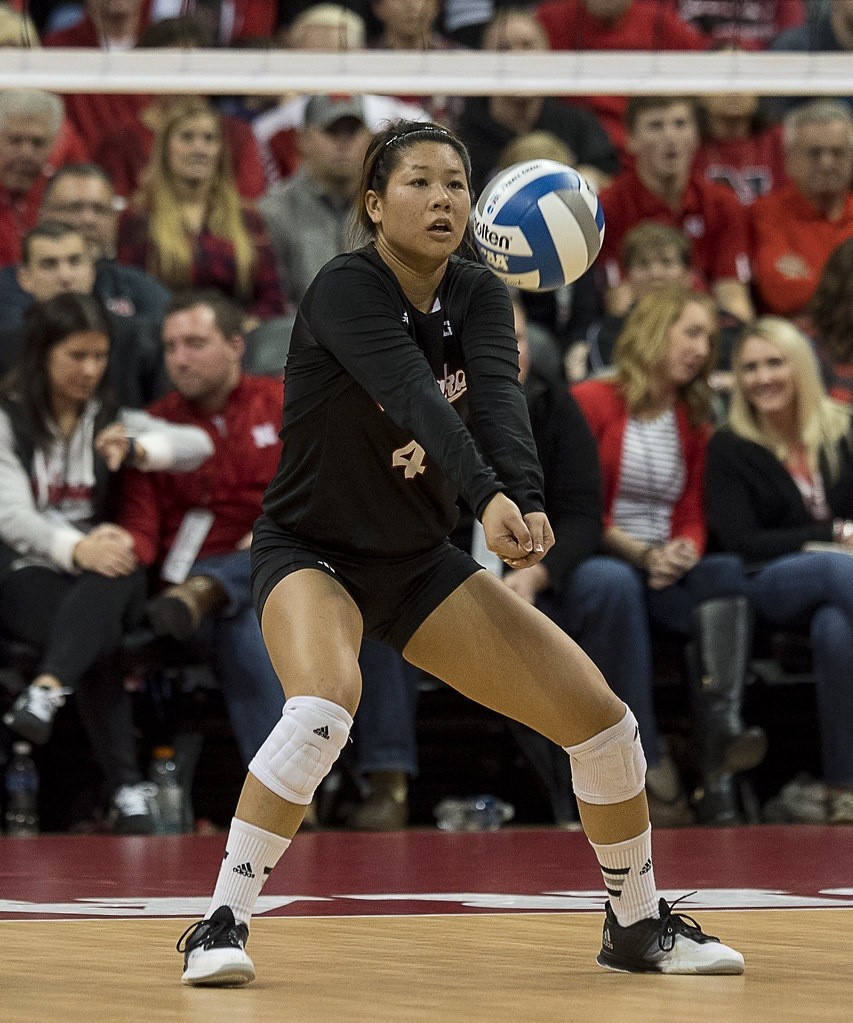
[692,597,767,782]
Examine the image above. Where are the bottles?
[7,742,38,838]
[436,795,515,831]
[151,747,193,835]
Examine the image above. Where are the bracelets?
[638,546,655,568]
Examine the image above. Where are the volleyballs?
[474,159,605,292]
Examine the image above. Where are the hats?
[301,88,370,128]
[440,0,495,32]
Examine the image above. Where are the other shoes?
[147,573,221,641]
[8,674,69,744]
[612,755,693,829]
[785,791,853,827]
[348,766,414,834]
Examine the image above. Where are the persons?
[176,119,746,985]
[0,0,853,834]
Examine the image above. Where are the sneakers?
[176,906,259,987]
[592,896,746,977]
